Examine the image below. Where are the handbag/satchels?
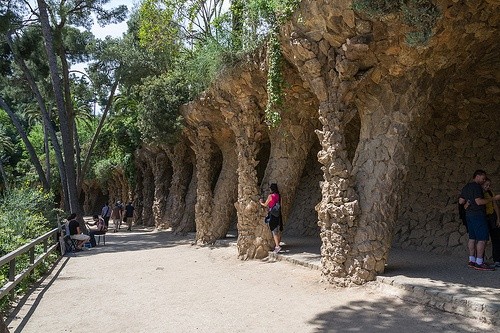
[269,202,281,217]
[123,217,127,222]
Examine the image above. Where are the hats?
[270,184,278,188]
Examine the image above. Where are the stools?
[70,238,81,250]
[97,233,106,246]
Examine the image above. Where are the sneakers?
[474,262,495,270]
[273,245,282,253]
[468,261,475,267]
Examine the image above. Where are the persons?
[123,202,138,231]
[65,216,72,236]
[259,183,283,253]
[111,203,121,233]
[69,213,91,249]
[458,169,500,270]
[458,178,500,267]
[85,215,107,247]
[102,202,111,231]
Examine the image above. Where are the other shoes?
[495,261,500,267]
